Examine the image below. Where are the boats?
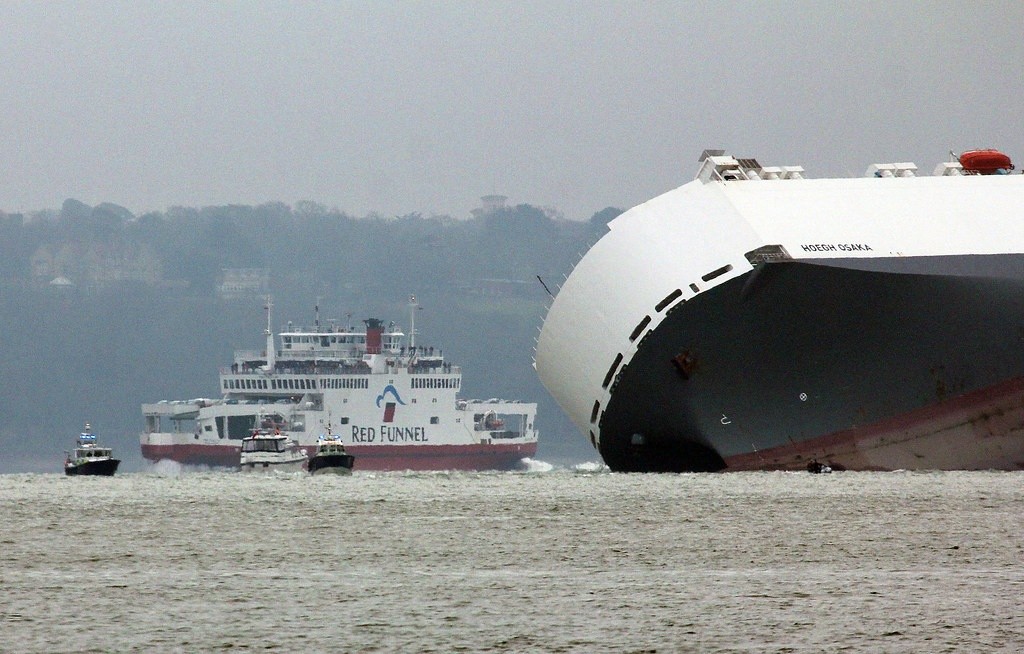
[64,422,121,476]
[531,143,1023,475]
[240,407,309,475]
[308,413,357,477]
[138,292,538,471]
